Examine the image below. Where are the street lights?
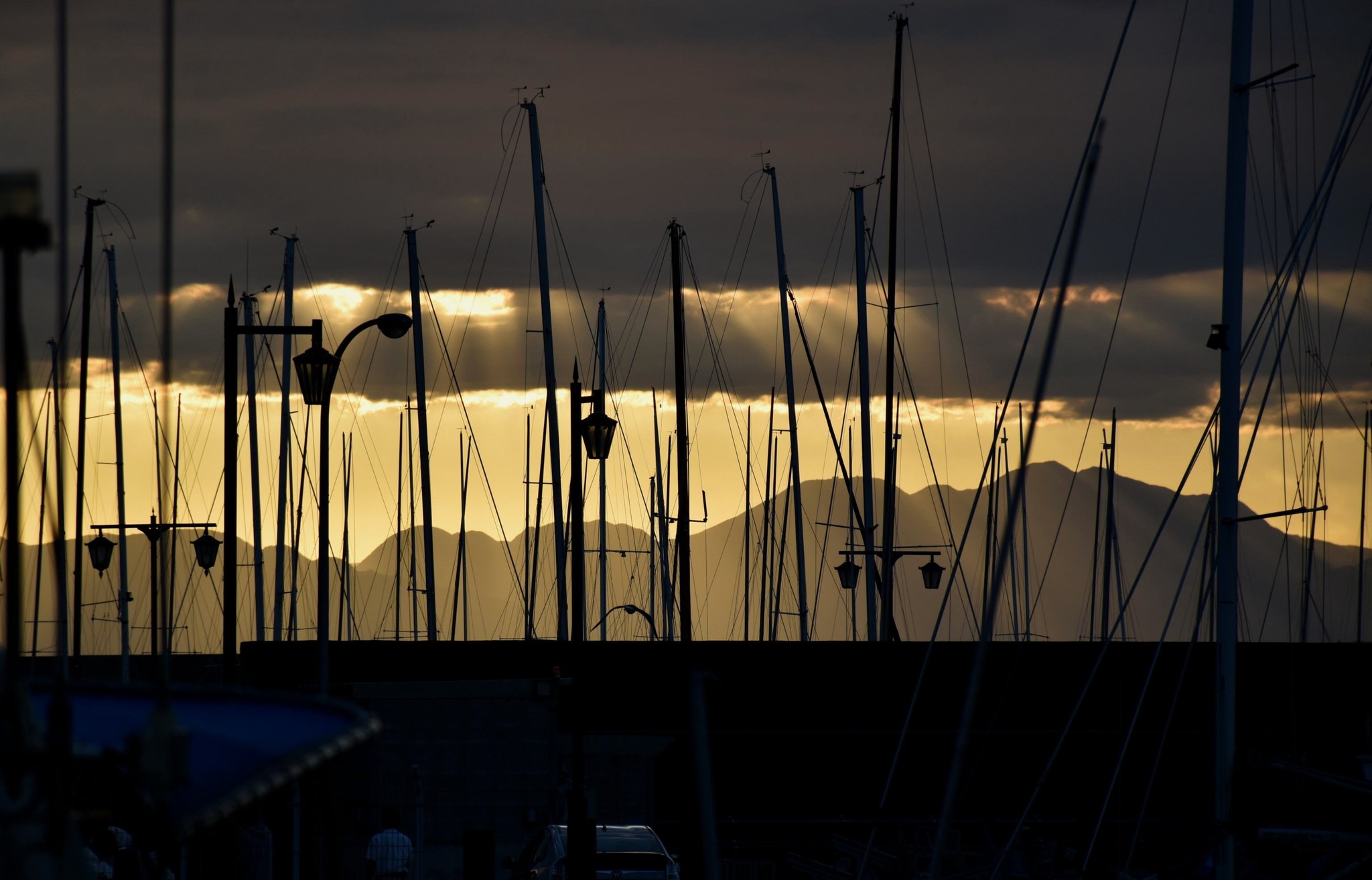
[226,274,344,658]
[317,311,413,684]
[569,354,618,640]
[835,549,947,642]
[84,506,224,653]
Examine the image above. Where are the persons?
[365,805,414,880]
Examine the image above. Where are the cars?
[523,824,681,880]
